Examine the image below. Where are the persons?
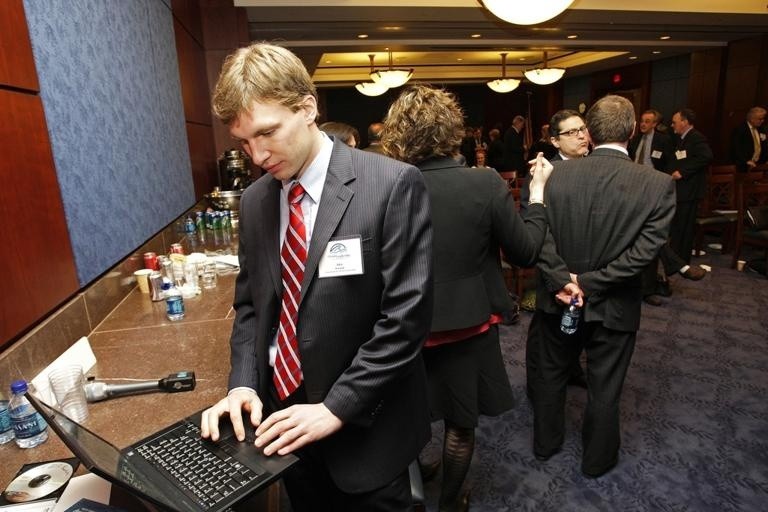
[199,41,438,512]
[518,95,680,478]
[377,78,555,512]
[318,107,768,391]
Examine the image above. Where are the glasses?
[548,124,588,138]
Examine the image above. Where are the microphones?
[87,372,198,400]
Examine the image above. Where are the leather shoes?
[458,495,470,512]
[642,295,663,306]
[582,456,618,479]
[678,262,706,281]
[421,459,441,481]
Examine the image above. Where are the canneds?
[214,211,219,228]
[219,212,225,229]
[159,259,176,285]
[208,213,216,228]
[165,296,185,321]
[223,210,230,227]
[170,244,186,256]
[156,255,168,270]
[195,212,204,230]
[144,252,155,269]
[205,212,210,227]
[147,272,165,302]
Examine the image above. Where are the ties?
[637,135,648,165]
[272,183,306,401]
[750,128,760,161]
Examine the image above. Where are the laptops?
[26,392,300,511]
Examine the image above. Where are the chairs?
[696,164,768,273]
[497,169,538,307]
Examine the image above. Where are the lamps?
[477,0,576,28]
[485,51,566,95]
[355,46,416,101]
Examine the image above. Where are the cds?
[4,460,73,502]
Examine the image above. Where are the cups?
[737,261,746,271]
[202,271,217,288]
[184,263,198,281]
[170,253,185,264]
[47,366,90,426]
[206,263,217,282]
[134,268,152,294]
[174,261,185,279]
[186,272,199,292]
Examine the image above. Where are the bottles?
[183,215,196,235]
[8,381,48,448]
[560,299,580,334]
[167,283,184,322]
[0,391,15,444]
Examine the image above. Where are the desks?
[2,212,286,508]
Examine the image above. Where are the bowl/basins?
[204,190,244,212]
